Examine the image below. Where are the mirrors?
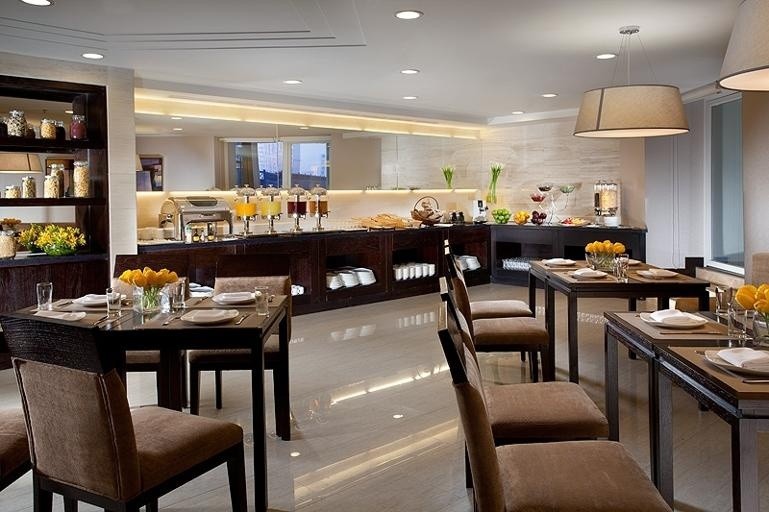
[135,100,484,191]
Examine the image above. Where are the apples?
[531,210,547,225]
[491,208,512,224]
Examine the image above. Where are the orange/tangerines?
[514,210,530,225]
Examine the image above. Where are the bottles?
[185,222,215,245]
[5,161,91,198]
[2,110,88,139]
[451,212,465,226]
[0,230,16,258]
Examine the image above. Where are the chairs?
[752,253,769,287]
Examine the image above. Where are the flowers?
[15,225,44,247]
[735,284,769,331]
[29,224,87,250]
[119,266,178,307]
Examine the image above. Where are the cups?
[728,309,747,336]
[255,286,269,315]
[616,254,629,283]
[502,256,542,270]
[106,292,121,318]
[715,287,732,316]
[291,284,304,296]
[172,280,185,308]
[36,282,53,310]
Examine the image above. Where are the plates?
[329,323,377,341]
[188,282,214,298]
[433,224,454,227]
[211,292,256,305]
[557,218,591,227]
[30,310,86,321]
[180,309,240,325]
[454,255,481,270]
[137,227,174,240]
[541,258,679,279]
[640,312,709,329]
[326,266,376,289]
[705,348,769,375]
[71,294,127,306]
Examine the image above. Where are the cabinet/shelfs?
[138,224,490,317]
[0,75,110,373]
[490,225,648,300]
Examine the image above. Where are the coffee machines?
[472,199,489,224]
[594,181,621,229]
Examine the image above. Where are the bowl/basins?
[398,312,435,328]
[393,262,435,281]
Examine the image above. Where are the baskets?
[411,196,441,225]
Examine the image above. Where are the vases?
[752,311,769,348]
[43,241,74,256]
[28,245,45,253]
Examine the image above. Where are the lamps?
[573,25,690,139]
[718,0,769,92]
